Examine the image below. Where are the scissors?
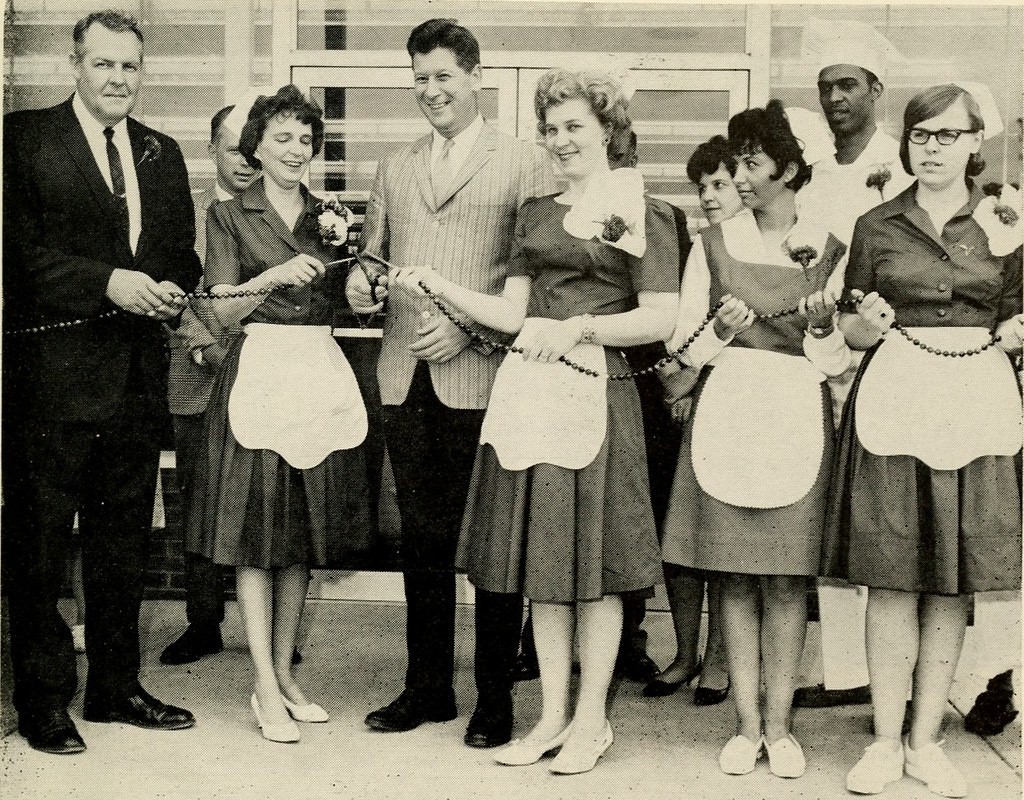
[352,248,388,310]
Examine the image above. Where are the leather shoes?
[83,674,196,731]
[19,709,86,753]
[464,687,514,747]
[365,686,459,732]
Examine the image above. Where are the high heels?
[643,652,705,698]
[493,717,572,765]
[694,666,731,704]
[280,693,328,724]
[251,686,300,743]
[550,719,613,774]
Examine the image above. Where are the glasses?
[905,127,975,145]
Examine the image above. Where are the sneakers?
[905,733,968,798]
[719,726,764,774]
[846,736,903,795]
[764,732,804,778]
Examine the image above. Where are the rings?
[300,274,308,282]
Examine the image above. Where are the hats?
[800,18,902,91]
[780,107,836,168]
[951,81,1005,141]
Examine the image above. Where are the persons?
[342,17,559,748]
[605,127,744,709]
[839,80,1024,798]
[158,83,385,744]
[793,19,916,739]
[390,70,681,775]
[665,98,850,778]
[2,9,203,755]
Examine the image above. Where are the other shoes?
[160,622,224,664]
[620,628,662,684]
[291,645,302,665]
[865,700,914,735]
[514,649,538,682]
[791,681,871,709]
[963,670,1018,734]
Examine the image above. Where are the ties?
[102,127,129,246]
[436,140,455,207]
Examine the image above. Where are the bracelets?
[810,324,834,334]
[880,312,886,319]
[579,314,596,346]
